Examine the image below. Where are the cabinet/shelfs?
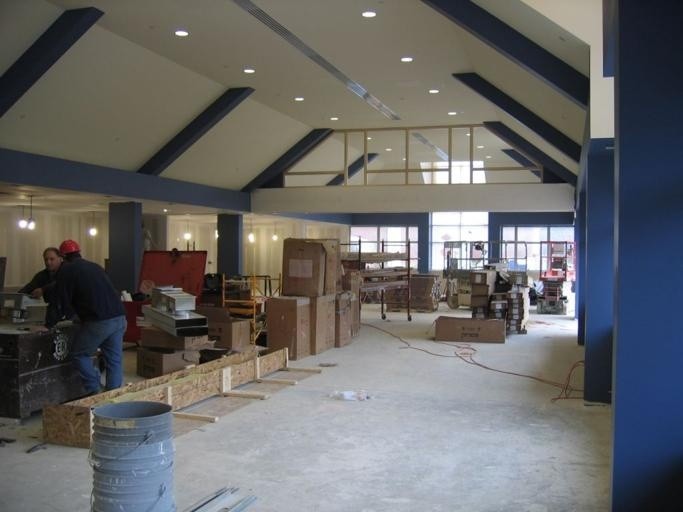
[336,238,413,322]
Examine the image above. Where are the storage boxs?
[266,237,360,360]
[136,304,255,381]
[468,268,530,334]
[119,250,207,344]
[433,316,506,345]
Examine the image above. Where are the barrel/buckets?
[87,401,176,511]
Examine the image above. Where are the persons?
[15,246,66,329]
[51,238,128,396]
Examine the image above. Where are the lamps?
[18,205,27,231]
[27,193,34,231]
[87,210,99,238]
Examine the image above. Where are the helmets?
[58,240,81,255]
